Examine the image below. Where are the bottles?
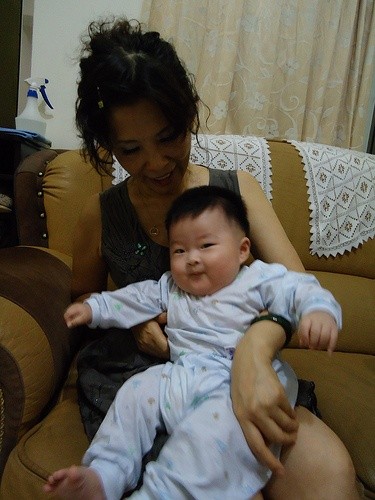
[15,75,53,140]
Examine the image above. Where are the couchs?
[0,136,375,500]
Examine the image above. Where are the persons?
[44,186,342,500]
[74,15,356,498]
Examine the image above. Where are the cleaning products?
[15,76,55,137]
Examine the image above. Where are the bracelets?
[250,313,293,350]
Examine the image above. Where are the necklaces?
[136,183,166,237]
